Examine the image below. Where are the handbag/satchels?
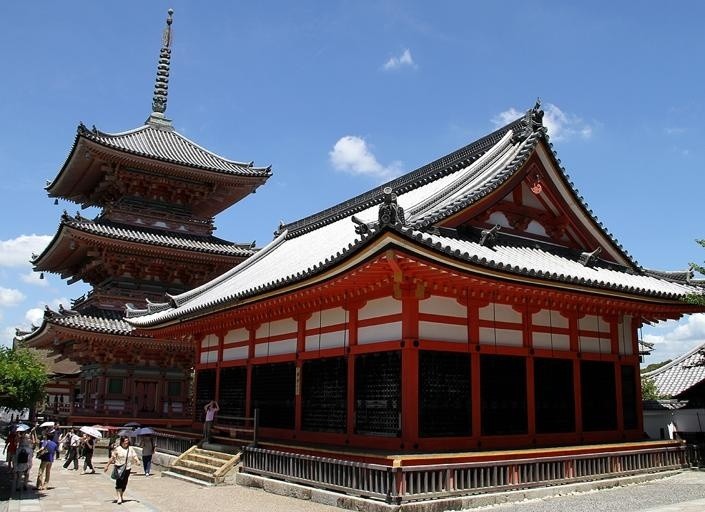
[36,449,49,459]
[152,447,154,453]
[81,448,86,456]
[110,464,125,479]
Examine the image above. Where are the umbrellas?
[79,422,154,439]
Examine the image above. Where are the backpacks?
[17,449,27,463]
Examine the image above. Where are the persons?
[43,392,64,403]
[104,436,141,504]
[3,422,96,500]
[109,430,155,476]
[203,400,220,441]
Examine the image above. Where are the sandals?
[117,498,123,504]
